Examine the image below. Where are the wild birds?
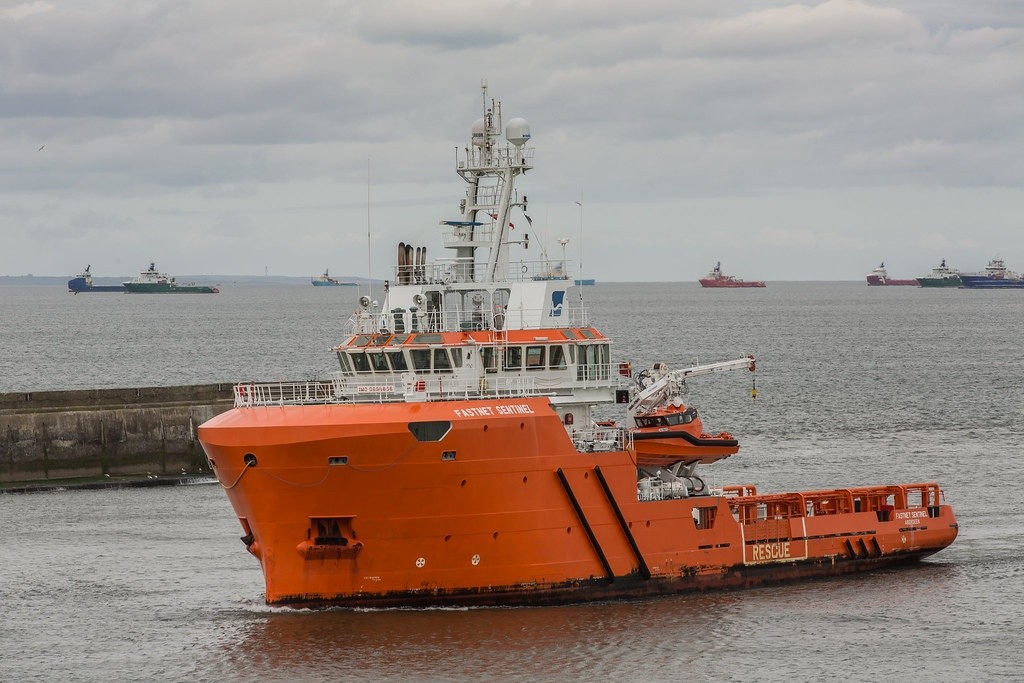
[39,144,47,151]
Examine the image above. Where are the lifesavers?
[521,265,528,273]
[479,376,489,392]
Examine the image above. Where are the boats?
[698,261,768,287]
[867,261,919,285]
[67,264,126,293]
[530,254,596,285]
[311,268,359,286]
[957,257,1024,288]
[915,258,963,287]
[122,262,220,293]
[190,80,959,611]
